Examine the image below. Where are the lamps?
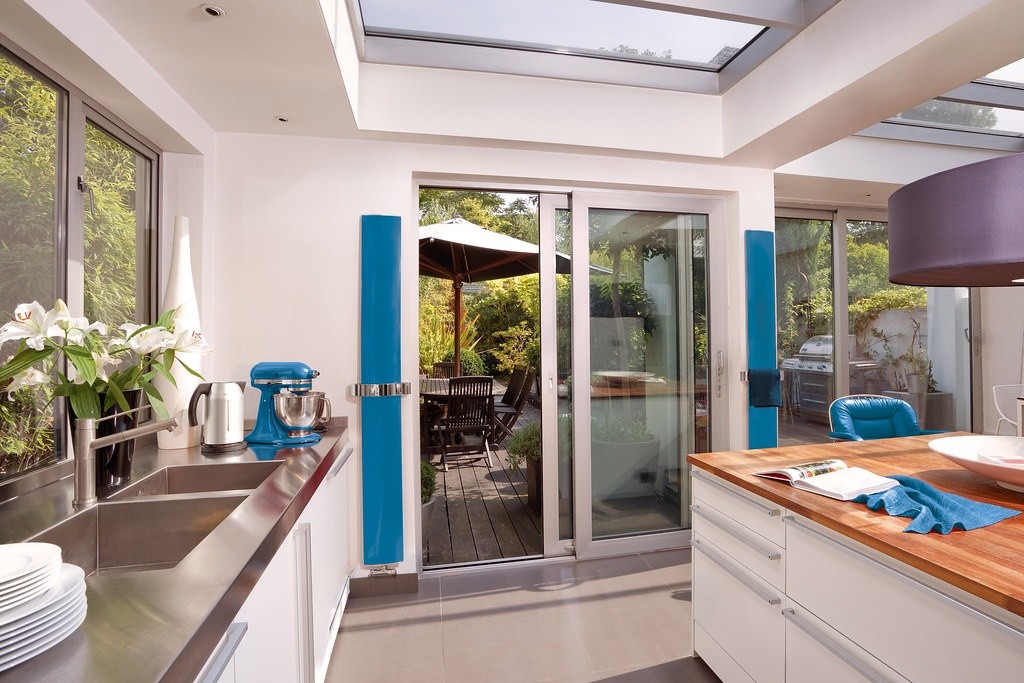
[887,152,1024,286]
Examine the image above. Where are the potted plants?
[420,460,440,565]
[504,208,675,519]
[879,317,954,431]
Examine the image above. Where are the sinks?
[105,460,285,500]
[927,435,1024,494]
[590,370,655,385]
[18,494,250,577]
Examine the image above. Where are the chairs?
[994,384,1024,435]
[421,361,536,472]
[828,392,949,443]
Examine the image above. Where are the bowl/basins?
[273,392,326,435]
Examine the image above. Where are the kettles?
[188,381,248,454]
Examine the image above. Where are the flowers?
[0,296,207,429]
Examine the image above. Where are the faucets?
[1016,397,1024,438]
[71,404,181,505]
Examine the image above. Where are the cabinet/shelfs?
[686,430,1024,683]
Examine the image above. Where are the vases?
[67,386,144,491]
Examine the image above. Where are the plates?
[927,435,1024,492]
[0,541,87,672]
[594,371,655,382]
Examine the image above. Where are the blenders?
[244,361,331,447]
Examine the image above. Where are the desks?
[422,386,503,453]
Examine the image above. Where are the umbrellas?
[418,213,613,377]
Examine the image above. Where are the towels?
[848,474,1023,535]
